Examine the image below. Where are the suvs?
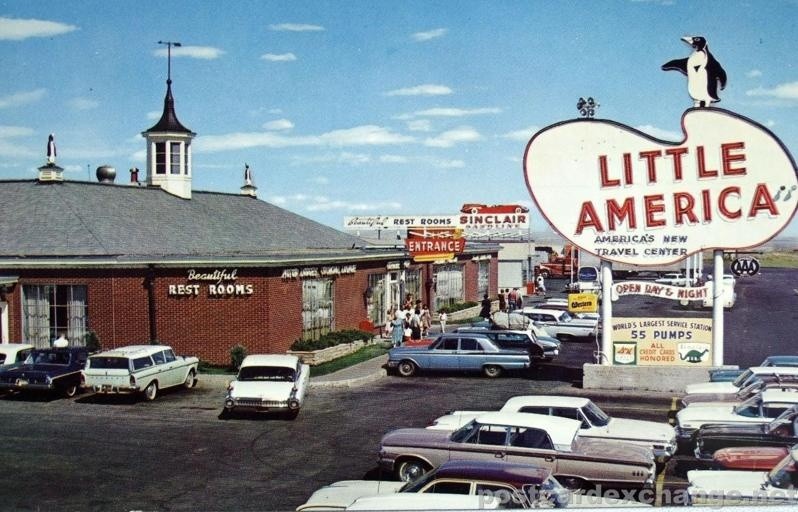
[80,341,201,402]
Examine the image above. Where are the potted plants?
[432,298,481,322]
[284,327,375,368]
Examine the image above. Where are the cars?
[291,458,660,511]
[651,272,694,287]
[0,344,98,398]
[439,293,599,361]
[220,354,312,414]
[373,411,661,492]
[0,343,35,367]
[670,349,797,507]
[386,332,532,382]
[532,253,577,279]
[338,487,503,512]
[425,390,681,465]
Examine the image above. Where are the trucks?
[700,273,737,313]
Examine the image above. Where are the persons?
[533,273,546,296]
[384,293,432,348]
[479,294,491,320]
[439,308,447,333]
[54,333,69,348]
[498,287,523,313]
